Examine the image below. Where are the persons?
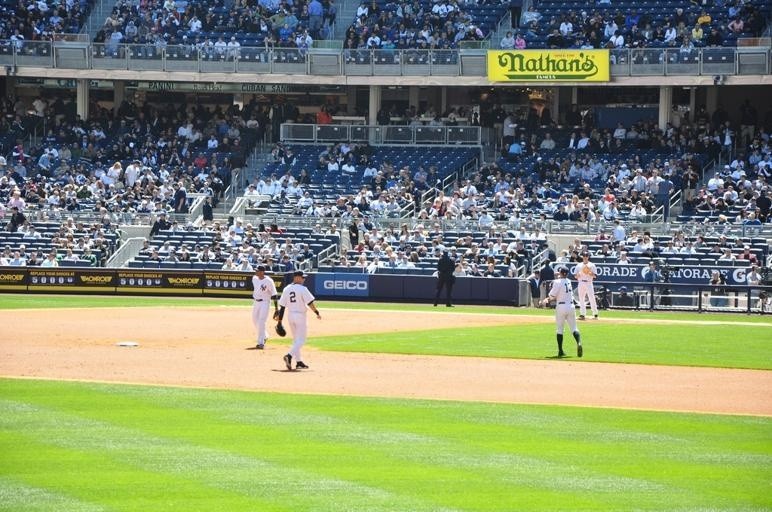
[278,271,321,370]
[0,78,771,309]
[1,1,770,68]
[251,265,278,349]
[574,252,598,318]
[539,266,582,358]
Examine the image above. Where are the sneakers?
[255,343,265,349]
[577,345,583,357]
[577,316,586,321]
[283,354,292,370]
[593,315,599,320]
[296,362,309,370]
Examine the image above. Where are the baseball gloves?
[273,310,281,320]
[275,323,287,336]
[583,266,591,276]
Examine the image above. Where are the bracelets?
[314,310,319,315]
[275,307,277,311]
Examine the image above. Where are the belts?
[580,280,590,282]
[254,298,264,301]
[559,301,575,305]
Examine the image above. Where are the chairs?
[1,1,772,314]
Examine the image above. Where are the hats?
[254,265,266,272]
[544,258,550,263]
[618,286,627,292]
[293,271,308,278]
[19,245,26,249]
[350,216,361,222]
[602,159,644,181]
[158,211,166,216]
[560,267,569,274]
[583,252,590,256]
